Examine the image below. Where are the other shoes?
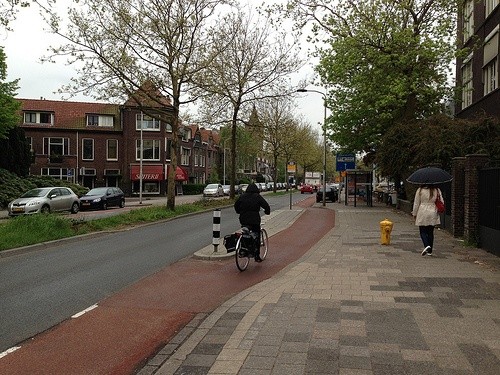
[255,256,262,262]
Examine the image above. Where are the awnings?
[131,164,189,181]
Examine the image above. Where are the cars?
[223,182,290,193]
[316,186,335,203]
[328,182,343,192]
[300,182,314,195]
[80,186,126,209]
[7,186,79,217]
[203,183,222,196]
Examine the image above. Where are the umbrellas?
[406,166,454,184]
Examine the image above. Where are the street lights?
[223,136,239,184]
[297,88,327,205]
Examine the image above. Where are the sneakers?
[427,252,432,255]
[421,246,431,256]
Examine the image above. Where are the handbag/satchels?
[435,188,444,213]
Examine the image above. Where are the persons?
[234,182,270,261]
[410,183,444,256]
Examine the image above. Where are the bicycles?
[236,209,269,272]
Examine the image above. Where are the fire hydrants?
[378,218,394,246]
